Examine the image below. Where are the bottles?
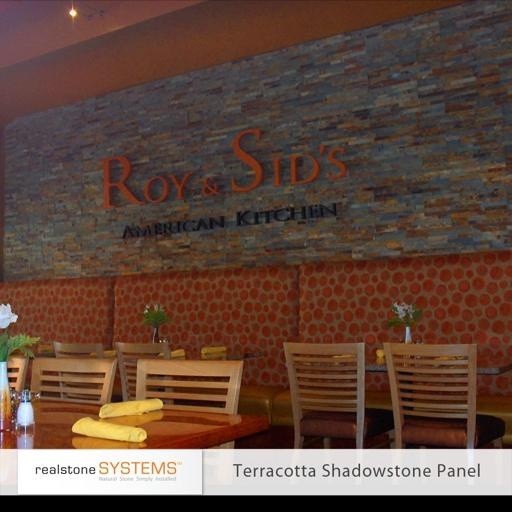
[15,389,35,426]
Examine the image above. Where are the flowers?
[143,304,172,325]
[0,303,41,362]
[387,298,421,327]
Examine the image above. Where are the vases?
[405,326,412,344]
[152,327,159,343]
